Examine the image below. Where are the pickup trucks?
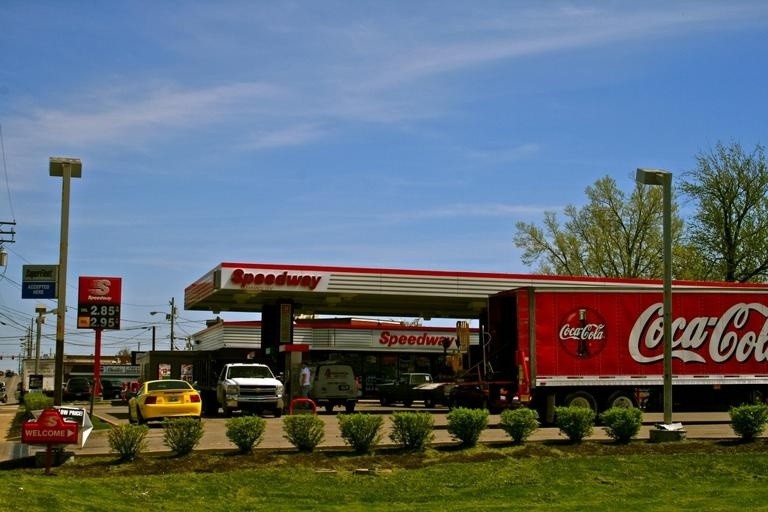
[375,371,457,409]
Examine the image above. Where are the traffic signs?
[22,410,77,444]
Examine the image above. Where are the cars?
[127,378,204,425]
[446,380,526,414]
[0,380,8,403]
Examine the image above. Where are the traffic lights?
[0,355,2,360]
[12,355,14,360]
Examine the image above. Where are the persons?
[355,375,359,403]
[299,361,312,410]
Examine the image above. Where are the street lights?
[34,307,45,359]
[47,155,83,406]
[633,167,688,445]
[150,297,176,350]
[0,244,8,267]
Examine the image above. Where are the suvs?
[304,359,361,414]
[215,362,287,417]
[64,376,92,401]
[101,379,123,398]
